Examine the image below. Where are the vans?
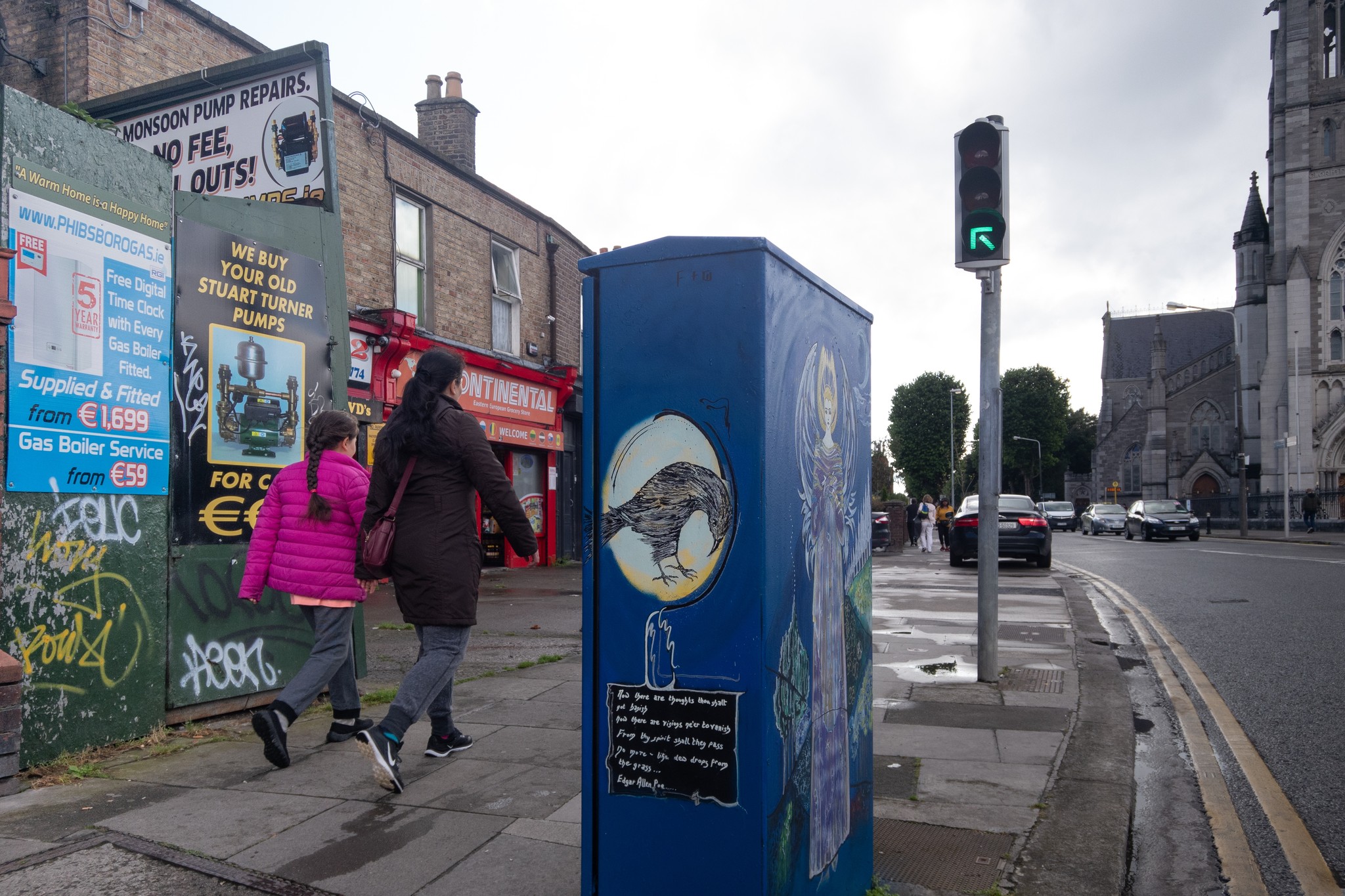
[1036,500,1078,532]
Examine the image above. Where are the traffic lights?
[953,117,1011,271]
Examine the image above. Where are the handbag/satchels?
[940,520,949,526]
[362,509,397,579]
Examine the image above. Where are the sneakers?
[251,706,291,768]
[353,724,406,795]
[424,725,473,758]
[326,717,375,743]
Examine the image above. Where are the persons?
[1123,503,1129,511]
[1301,488,1322,534]
[906,497,922,546]
[936,498,955,551]
[238,410,378,766]
[917,494,936,552]
[354,350,539,794]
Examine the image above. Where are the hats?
[942,497,948,501]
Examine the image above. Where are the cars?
[1124,499,1200,542]
[871,511,891,550]
[945,492,1053,569]
[1080,502,1127,536]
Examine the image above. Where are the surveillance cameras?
[545,315,555,323]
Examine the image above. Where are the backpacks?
[918,502,930,519]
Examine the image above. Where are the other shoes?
[922,548,926,552]
[1307,527,1314,534]
[914,541,918,545]
[928,551,931,552]
[910,544,914,546]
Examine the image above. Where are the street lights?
[1013,436,1043,502]
[950,388,962,513]
[1166,302,1249,538]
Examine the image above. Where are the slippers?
[940,547,945,551]
[945,548,950,552]
